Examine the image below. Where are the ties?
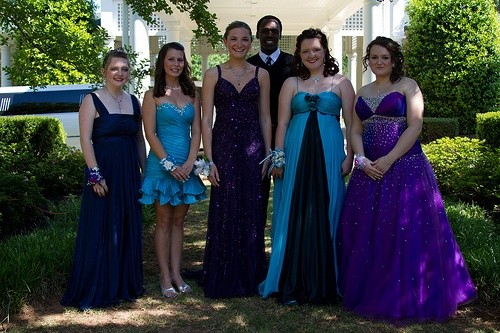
[265,57,272,66]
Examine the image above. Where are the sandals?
[161,287,177,299]
[171,279,192,294]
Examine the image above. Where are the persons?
[201,20,272,300]
[257,25,354,307]
[138,42,209,299]
[246,14,310,261]
[334,36,480,327]
[60,50,147,314]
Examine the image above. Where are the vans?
[0,83,103,181]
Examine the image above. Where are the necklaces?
[104,85,124,113]
[165,83,182,97]
[308,75,322,84]
[227,63,247,88]
[373,83,388,93]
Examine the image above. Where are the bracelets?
[84,166,103,187]
[193,159,214,178]
[159,154,179,174]
[383,157,392,167]
[354,155,366,170]
[259,144,286,179]
